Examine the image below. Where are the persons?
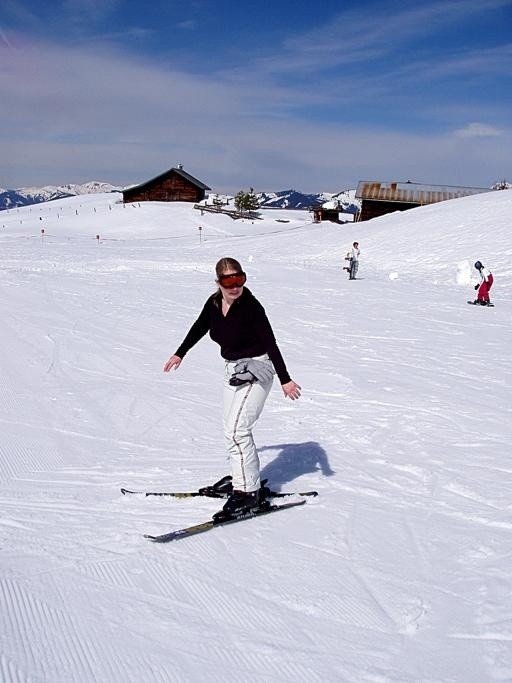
[475,261,494,305]
[347,242,360,280]
[163,257,302,521]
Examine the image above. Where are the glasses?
[217,271,246,289]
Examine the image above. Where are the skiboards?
[467,299,494,306]
[121,489,318,540]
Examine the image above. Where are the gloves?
[229,358,275,386]
[475,284,480,290]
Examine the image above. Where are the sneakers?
[224,489,264,512]
[213,476,234,491]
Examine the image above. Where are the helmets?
[476,262,481,269]
